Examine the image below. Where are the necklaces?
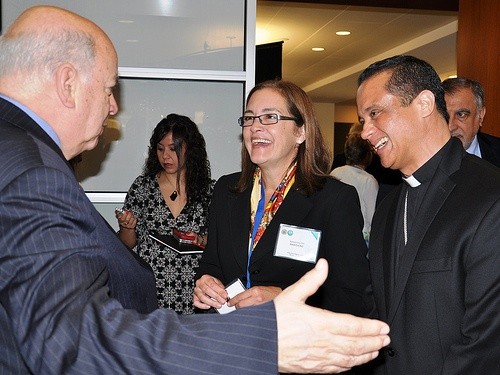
[162,170,185,202]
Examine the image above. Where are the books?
[148,234,204,254]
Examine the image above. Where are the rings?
[124,223,129,225]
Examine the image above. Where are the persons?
[354,56,500,375]
[191,79,380,334]
[114,113,215,317]
[327,123,381,257]
[0,5,392,375]
[439,75,499,170]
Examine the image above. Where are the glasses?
[238,113,298,127]
